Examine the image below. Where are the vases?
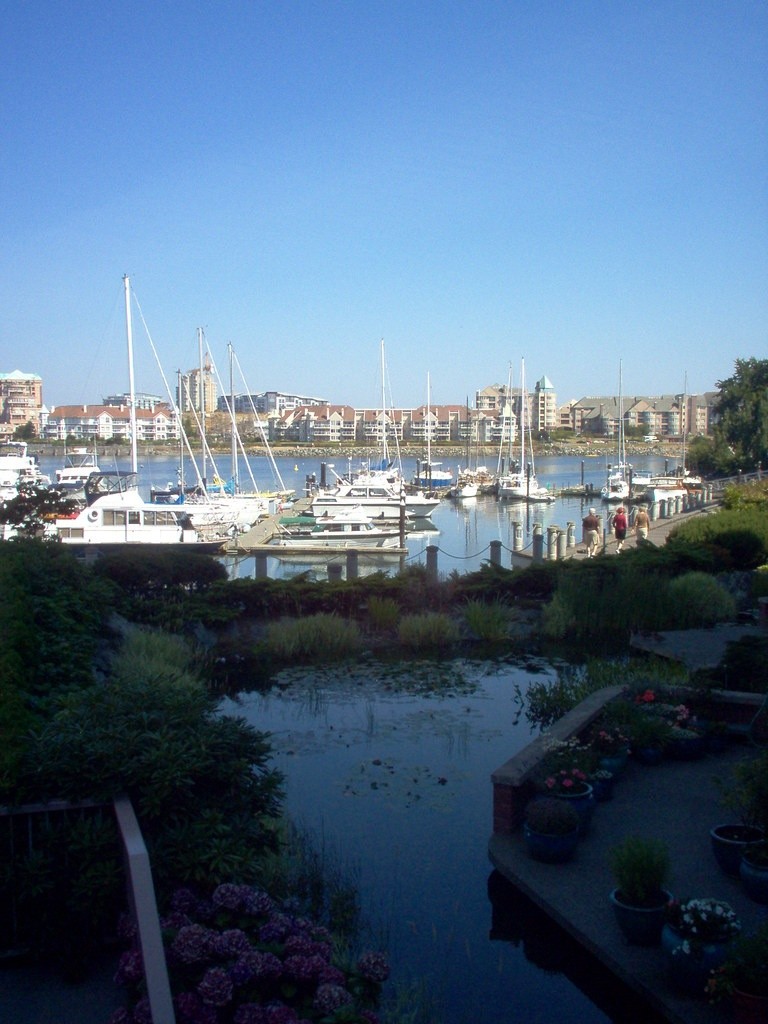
[535,782,596,822]
[593,779,612,801]
[663,736,694,760]
[599,752,628,778]
[635,742,662,765]
[661,921,708,977]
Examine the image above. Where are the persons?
[631,507,649,548]
[612,507,627,554]
[583,507,600,559]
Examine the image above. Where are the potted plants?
[606,829,674,946]
[706,749,768,905]
[722,920,768,1024]
[524,799,581,862]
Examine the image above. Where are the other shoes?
[615,550,620,554]
[591,553,595,559]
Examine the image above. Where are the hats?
[617,508,625,512]
[639,507,645,512]
[589,508,596,514]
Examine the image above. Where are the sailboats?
[1,274,705,565]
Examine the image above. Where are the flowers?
[701,963,730,1003]
[669,897,740,956]
[541,767,586,794]
[541,688,701,778]
